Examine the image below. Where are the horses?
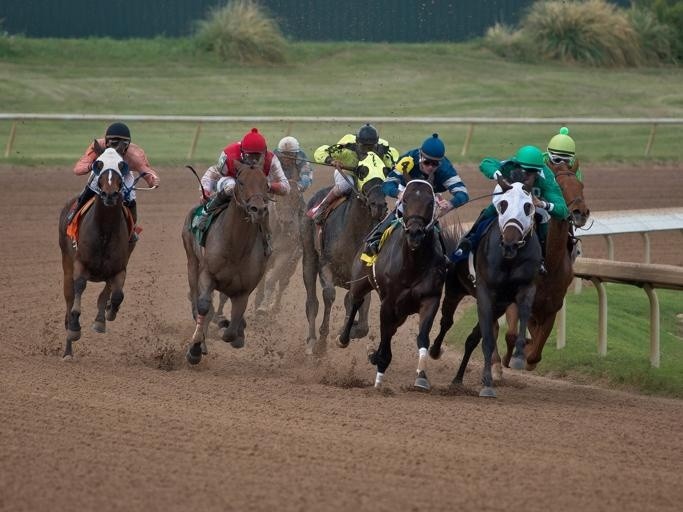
[490,158,589,382]
[59,139,136,362]
[335,166,447,391]
[300,134,388,359]
[428,173,542,398]
[253,149,306,317]
[181,147,273,365]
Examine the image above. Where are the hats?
[546,135,576,157]
[419,133,445,160]
[512,146,544,172]
[240,127,267,155]
[356,124,379,145]
[277,137,300,153]
[105,122,130,142]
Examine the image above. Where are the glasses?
[422,160,439,166]
[547,151,573,165]
[107,140,129,146]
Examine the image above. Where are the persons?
[542,126,583,259]
[272,136,313,203]
[367,132,469,263]
[308,123,399,223]
[450,145,569,277]
[73,122,160,245]
[198,127,290,233]
